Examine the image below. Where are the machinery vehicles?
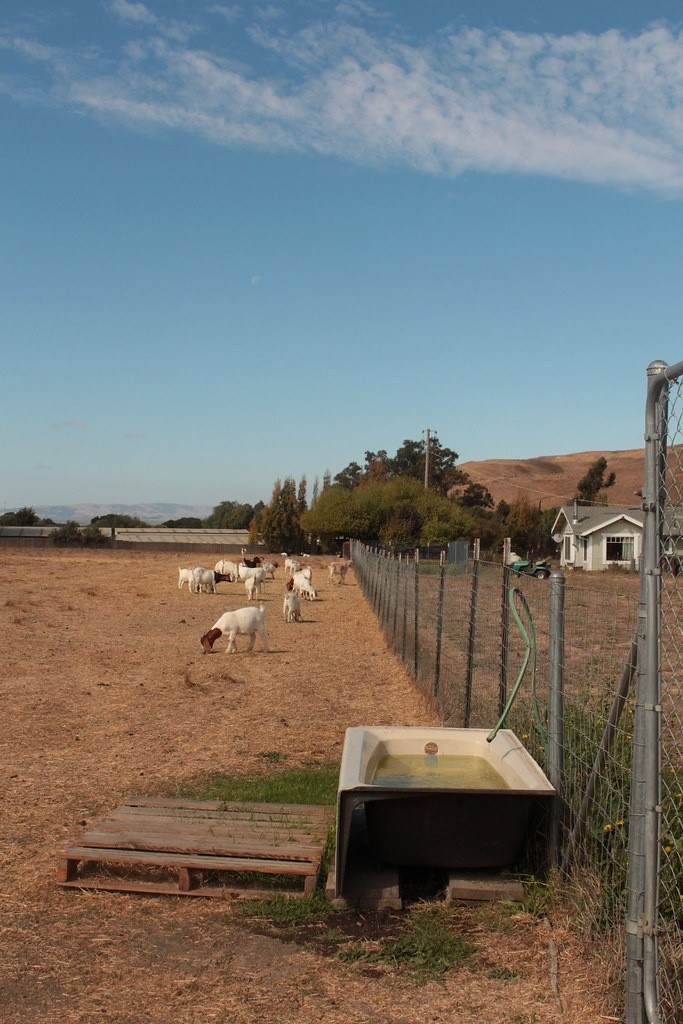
[510,559,552,580]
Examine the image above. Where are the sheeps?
[177,552,353,654]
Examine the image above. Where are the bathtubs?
[335,725,556,897]
